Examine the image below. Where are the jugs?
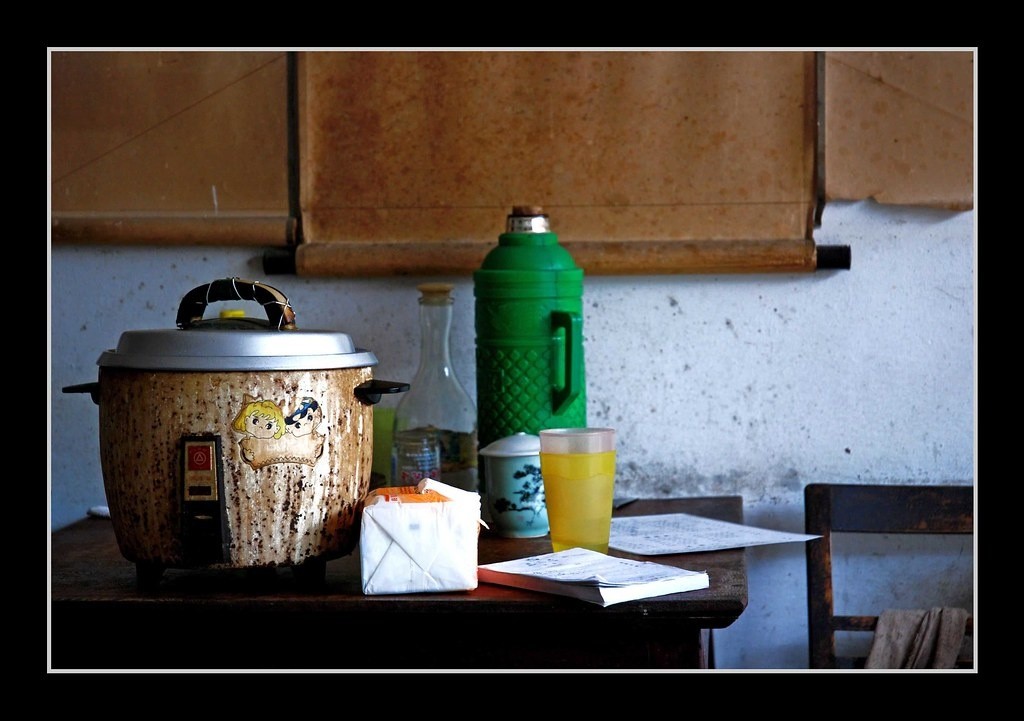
[472,204,588,525]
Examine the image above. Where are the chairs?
[804,482,974,668]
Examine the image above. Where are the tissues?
[360,477,489,599]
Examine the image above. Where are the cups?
[480,431,549,540]
[538,425,615,553]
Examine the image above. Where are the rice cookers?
[61,276,412,585]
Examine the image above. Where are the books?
[478,547,710,607]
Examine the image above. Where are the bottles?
[391,284,480,493]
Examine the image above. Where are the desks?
[51,498,748,669]
